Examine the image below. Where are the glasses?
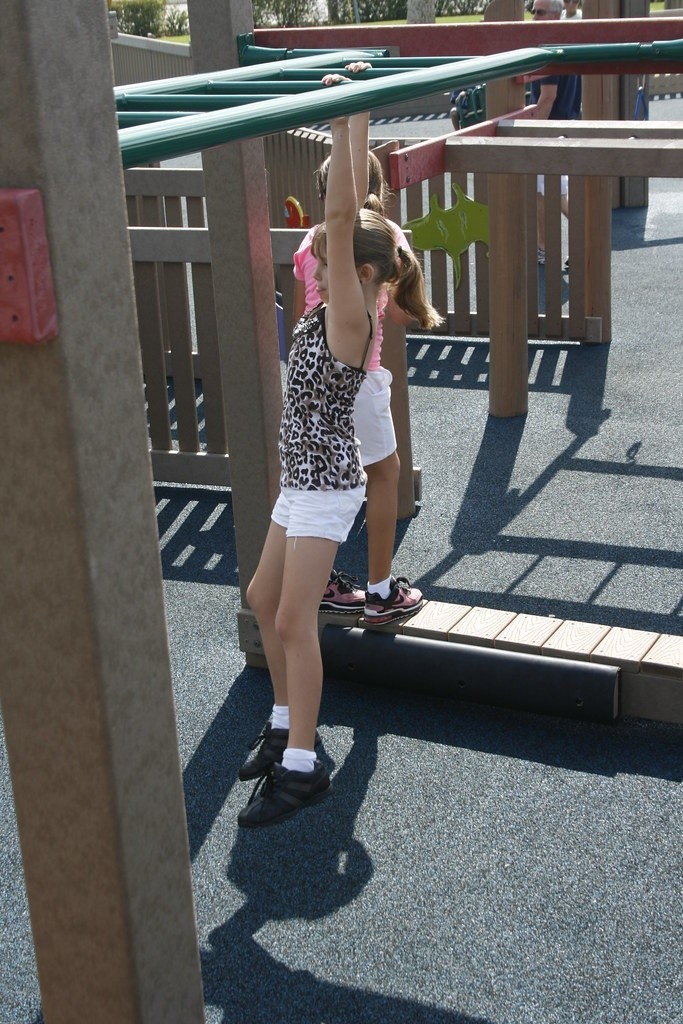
[564,0,580,4]
[531,8,547,16]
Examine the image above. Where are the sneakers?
[535,247,547,265]
[318,568,366,614]
[238,722,322,782]
[363,576,423,626]
[566,256,571,267]
[236,760,334,828]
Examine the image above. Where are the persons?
[527,0,580,266]
[293,152,423,629]
[450,86,478,133]
[559,0,582,20]
[236,61,444,827]
